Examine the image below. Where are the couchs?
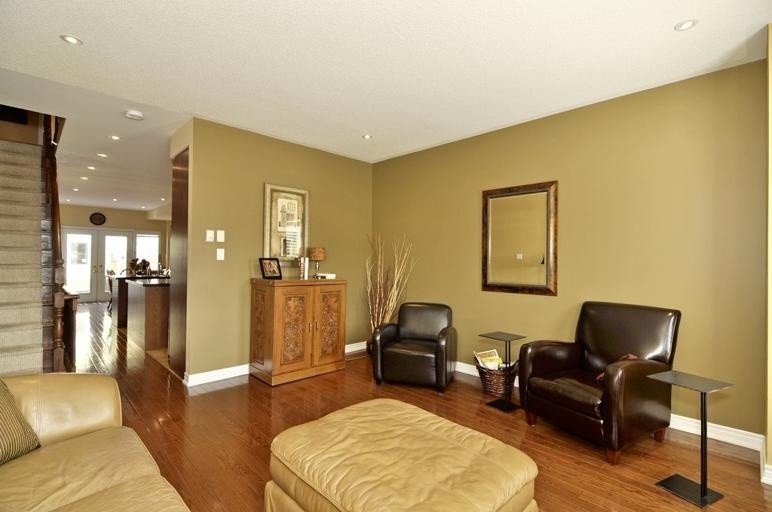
[2,371,193,512]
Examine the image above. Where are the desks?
[477,329,527,416]
[642,368,736,507]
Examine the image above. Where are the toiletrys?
[300,256,309,281]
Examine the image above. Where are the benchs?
[261,397,539,512]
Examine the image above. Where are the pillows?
[595,353,637,384]
[2,382,43,466]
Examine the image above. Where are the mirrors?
[480,179,561,297]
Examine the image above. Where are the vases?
[365,333,373,357]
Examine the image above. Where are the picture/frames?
[258,256,283,280]
[262,181,310,269]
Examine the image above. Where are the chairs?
[371,300,459,393]
[514,299,683,466]
[106,276,113,313]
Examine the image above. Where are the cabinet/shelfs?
[248,275,349,387]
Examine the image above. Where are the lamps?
[306,245,328,280]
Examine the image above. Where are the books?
[473,349,518,370]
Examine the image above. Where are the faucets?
[157,262,163,274]
[146,268,151,277]
[119,268,133,275]
